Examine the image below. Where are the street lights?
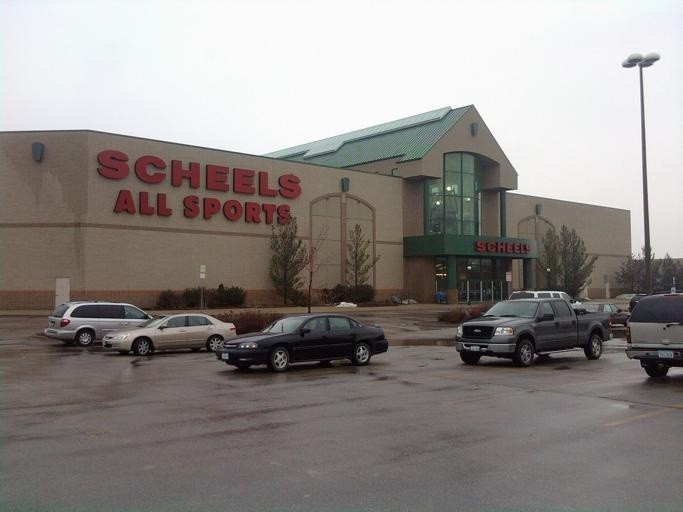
[622,52,662,295]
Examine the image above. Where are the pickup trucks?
[456,297,613,368]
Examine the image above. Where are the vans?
[507,290,586,315]
[624,292,683,378]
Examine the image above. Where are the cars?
[580,301,632,327]
[628,293,648,313]
[215,313,388,373]
[43,299,154,348]
[615,293,646,300]
[101,313,236,356]
